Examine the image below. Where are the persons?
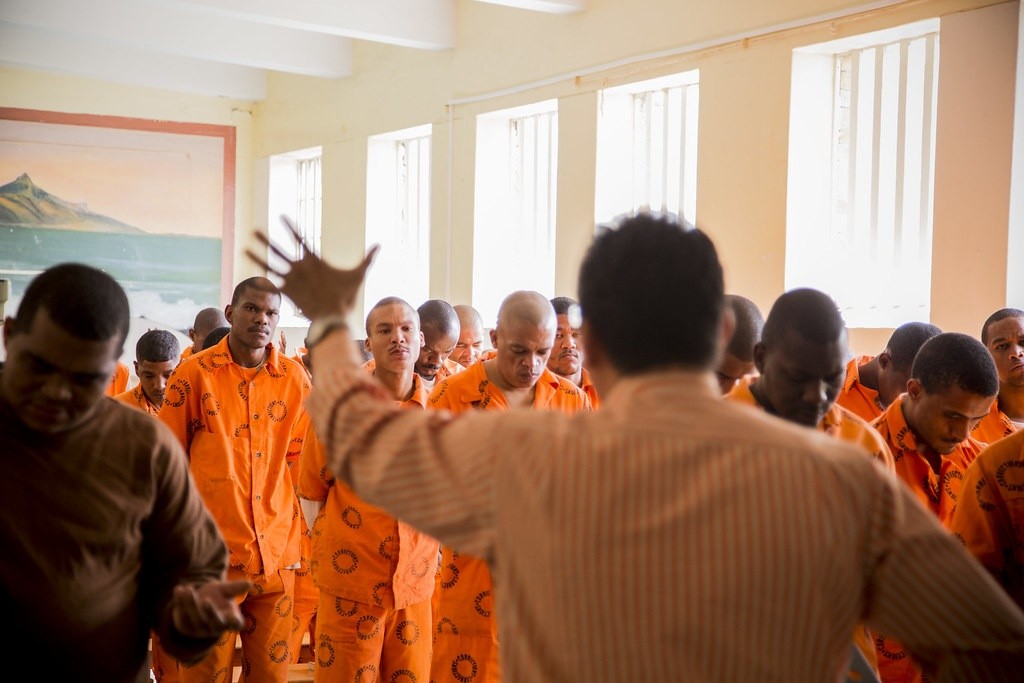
[427,291,590,683]
[0,263,252,683]
[109,328,180,682]
[725,287,892,679]
[199,323,317,680]
[300,297,435,681]
[159,277,314,683]
[833,320,942,423]
[864,330,1001,682]
[176,305,229,360]
[715,287,762,395]
[971,302,1021,445]
[449,301,491,368]
[247,211,1021,682]
[548,298,602,410]
